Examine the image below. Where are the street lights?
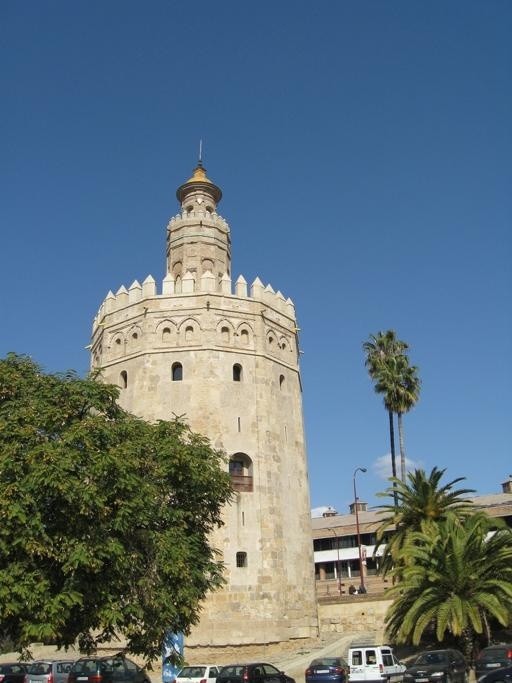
[328,528,341,596]
[354,467,366,593]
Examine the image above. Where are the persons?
[338,582,366,595]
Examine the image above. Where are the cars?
[0,657,150,683]
